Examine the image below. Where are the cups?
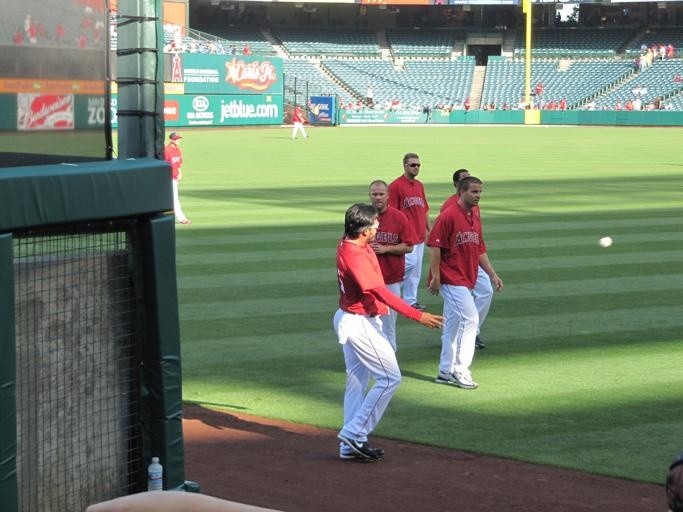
[183,478,200,492]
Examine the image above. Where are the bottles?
[147,454,163,495]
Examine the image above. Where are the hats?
[169,132,182,141]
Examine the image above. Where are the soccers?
[600,236,612,248]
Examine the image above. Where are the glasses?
[404,163,421,167]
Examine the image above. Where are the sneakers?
[475,336,485,349]
[435,368,478,389]
[180,219,191,224]
[337,433,385,462]
[411,303,427,310]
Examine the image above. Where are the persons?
[165,132,191,225]
[292,105,309,139]
[366,180,414,354]
[387,153,433,312]
[665,453,683,512]
[426,177,503,389]
[334,202,446,464]
[439,169,494,349]
